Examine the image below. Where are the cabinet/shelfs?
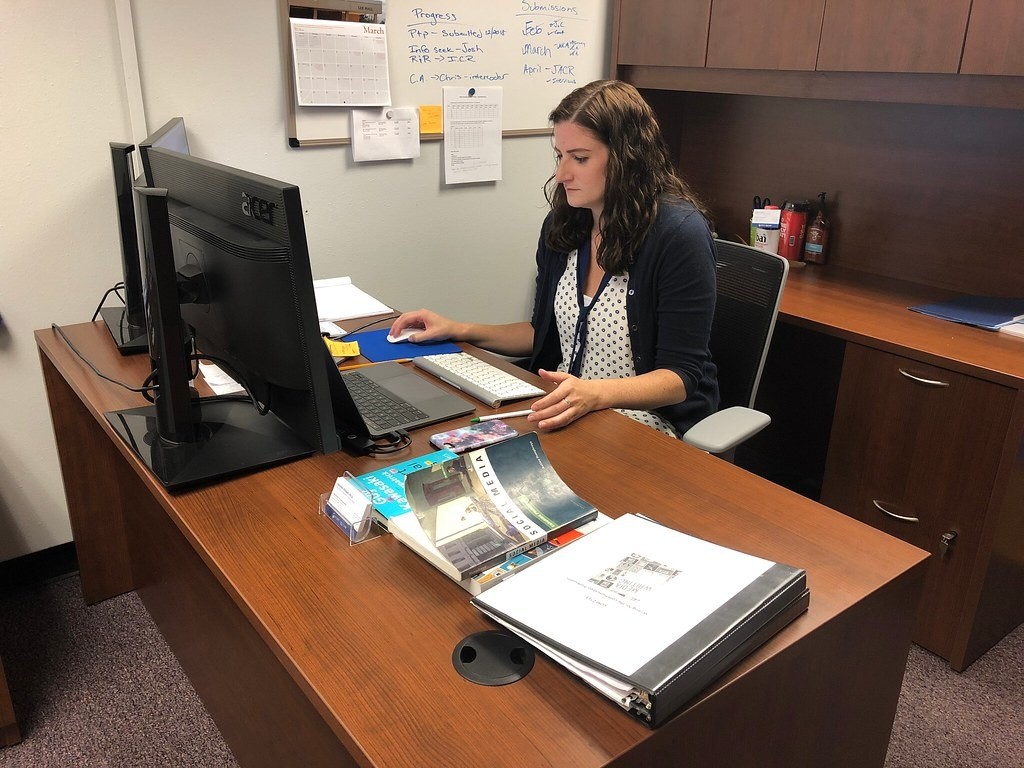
[614,0,1024,113]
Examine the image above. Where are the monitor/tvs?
[97,118,341,493]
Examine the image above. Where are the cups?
[750,219,780,256]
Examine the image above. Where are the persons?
[389,80,720,442]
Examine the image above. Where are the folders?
[471,508,811,730]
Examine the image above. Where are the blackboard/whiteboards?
[279,1,613,147]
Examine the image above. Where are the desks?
[34,289,933,767]
[716,259,1024,673]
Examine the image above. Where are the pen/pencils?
[338,358,413,371]
[470,409,536,423]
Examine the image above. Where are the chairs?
[682,238,791,454]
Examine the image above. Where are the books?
[312,277,395,320]
[350,432,810,730]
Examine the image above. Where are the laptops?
[321,339,476,441]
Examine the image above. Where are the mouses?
[386,328,427,343]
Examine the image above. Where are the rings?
[563,397,571,408]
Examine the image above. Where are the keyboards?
[412,352,548,409]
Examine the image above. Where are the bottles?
[777,200,812,260]
